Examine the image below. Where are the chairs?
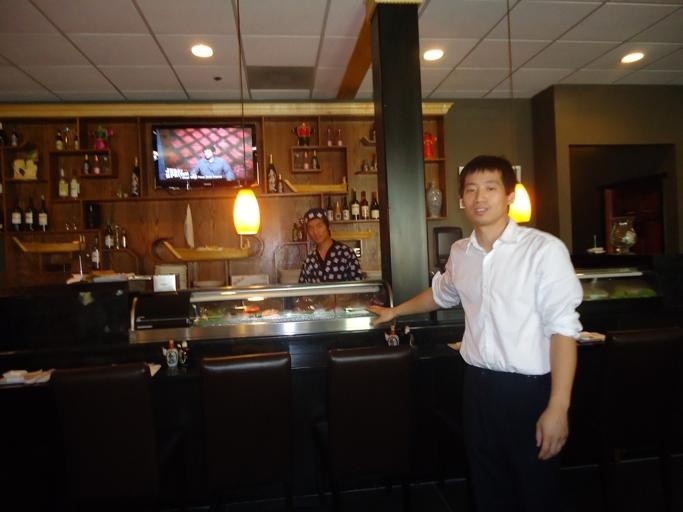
[198,350,295,508]
[46,361,156,510]
[430,341,473,511]
[606,325,682,504]
[312,345,416,509]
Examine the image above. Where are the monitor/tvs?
[432,226,464,265]
[153,121,260,192]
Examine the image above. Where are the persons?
[190,146,236,180]
[299,207,362,284]
[364,155,584,511]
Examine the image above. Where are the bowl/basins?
[2,369,28,384]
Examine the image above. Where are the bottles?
[10,191,48,232]
[291,191,380,242]
[90,218,128,271]
[0,121,20,147]
[424,131,438,156]
[54,122,140,197]
[166,337,188,368]
[268,121,344,192]
[362,150,377,171]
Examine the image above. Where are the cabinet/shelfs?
[261,118,446,222]
[575,267,656,316]
[129,282,396,344]
[1,116,115,255]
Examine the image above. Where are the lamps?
[503,1,531,224]
[230,66,262,236]
